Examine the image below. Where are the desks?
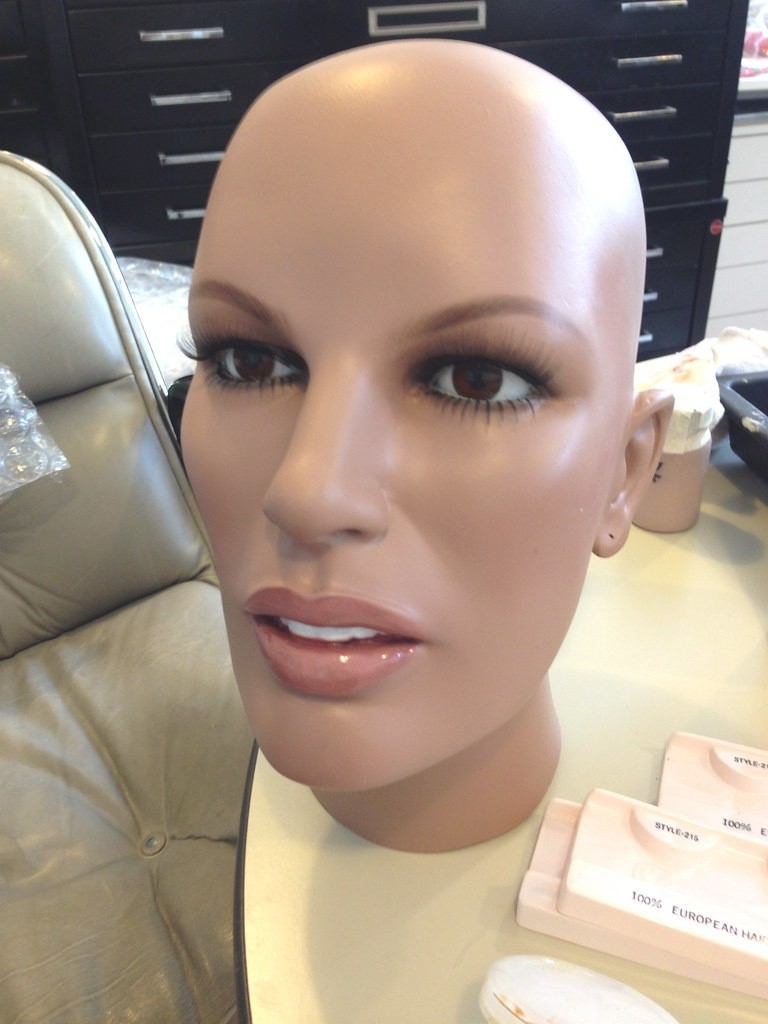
[232,350,768,1024]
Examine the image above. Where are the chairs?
[0,148,260,1024]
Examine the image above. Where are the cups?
[625,424,712,531]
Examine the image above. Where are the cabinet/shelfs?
[0,0,748,363]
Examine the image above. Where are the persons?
[179,38,675,853]
